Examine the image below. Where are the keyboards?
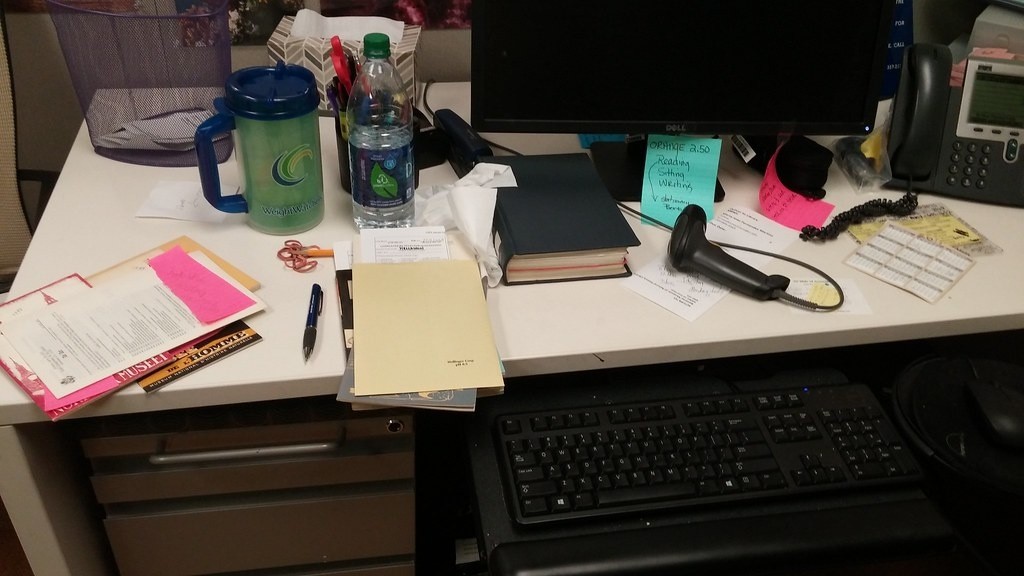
[494,382,923,532]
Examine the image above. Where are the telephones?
[883,43,1024,208]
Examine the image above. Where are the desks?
[0,43,1024,576]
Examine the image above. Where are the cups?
[334,110,420,195]
[194,60,324,235]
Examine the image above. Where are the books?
[474,151,641,286]
[331,224,506,414]
[0,235,268,423]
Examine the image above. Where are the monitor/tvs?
[469,0,899,203]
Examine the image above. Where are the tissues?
[269,8,420,116]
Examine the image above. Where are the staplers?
[434,110,493,170]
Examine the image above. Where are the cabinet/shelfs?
[79,396,417,576]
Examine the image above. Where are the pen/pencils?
[303,283,325,361]
[330,36,355,96]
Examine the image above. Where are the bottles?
[347,33,414,232]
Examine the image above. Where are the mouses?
[969,377,1024,448]
